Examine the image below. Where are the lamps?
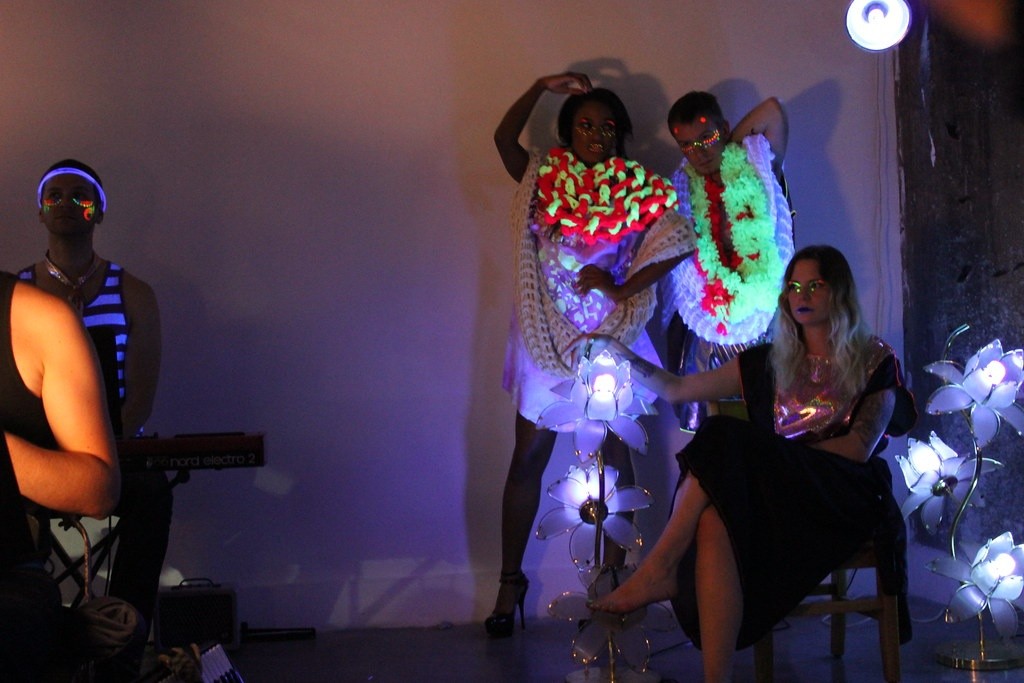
[523,336,666,683]
[896,321,1024,672]
[843,0,912,53]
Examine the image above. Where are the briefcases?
[153,577,239,651]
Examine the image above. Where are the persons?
[0,159,173,682]
[484,71,918,683]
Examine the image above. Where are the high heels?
[485,571,528,636]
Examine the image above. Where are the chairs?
[709,398,901,683]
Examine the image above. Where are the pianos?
[114,431,266,472]
